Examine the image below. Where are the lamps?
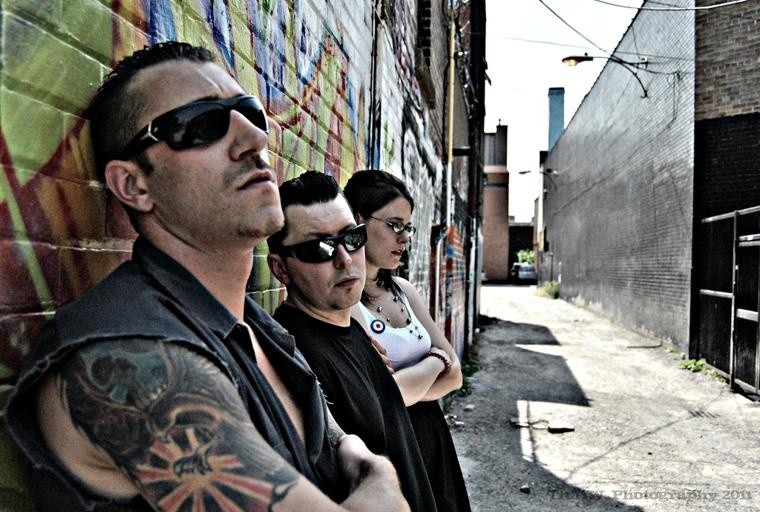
[561,52,651,101]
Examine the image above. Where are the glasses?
[369,215,416,238]
[114,93,270,161]
[277,223,367,264]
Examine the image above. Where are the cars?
[481,269,489,282]
[511,262,538,281]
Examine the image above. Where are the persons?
[344,170,472,512]
[267,170,437,512]
[3,41,411,512]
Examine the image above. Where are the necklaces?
[362,278,422,339]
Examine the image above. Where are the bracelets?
[420,351,450,379]
